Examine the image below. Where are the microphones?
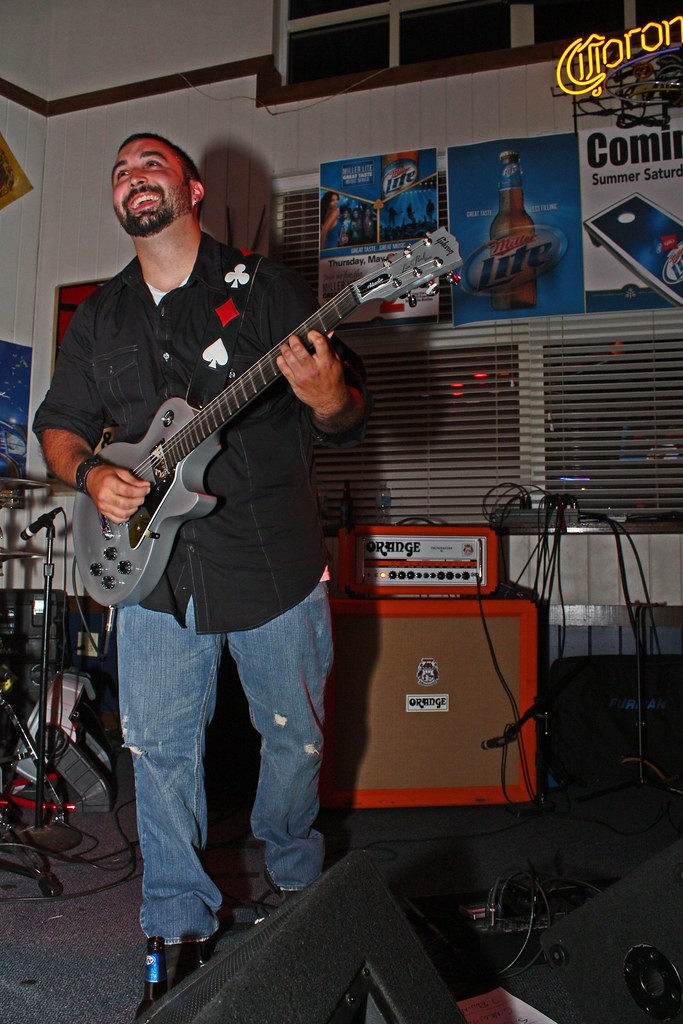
[481,732,517,750]
[20,507,63,541]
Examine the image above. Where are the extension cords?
[489,509,580,528]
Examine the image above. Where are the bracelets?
[75,456,108,494]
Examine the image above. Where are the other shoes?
[263,864,299,906]
[133,929,221,1022]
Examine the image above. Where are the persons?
[37,135,375,1017]
[324,192,373,247]
[381,200,437,239]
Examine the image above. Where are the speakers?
[537,838,683,1024]
[133,852,467,1024]
[318,598,539,811]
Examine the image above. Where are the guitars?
[71,226,467,609]
